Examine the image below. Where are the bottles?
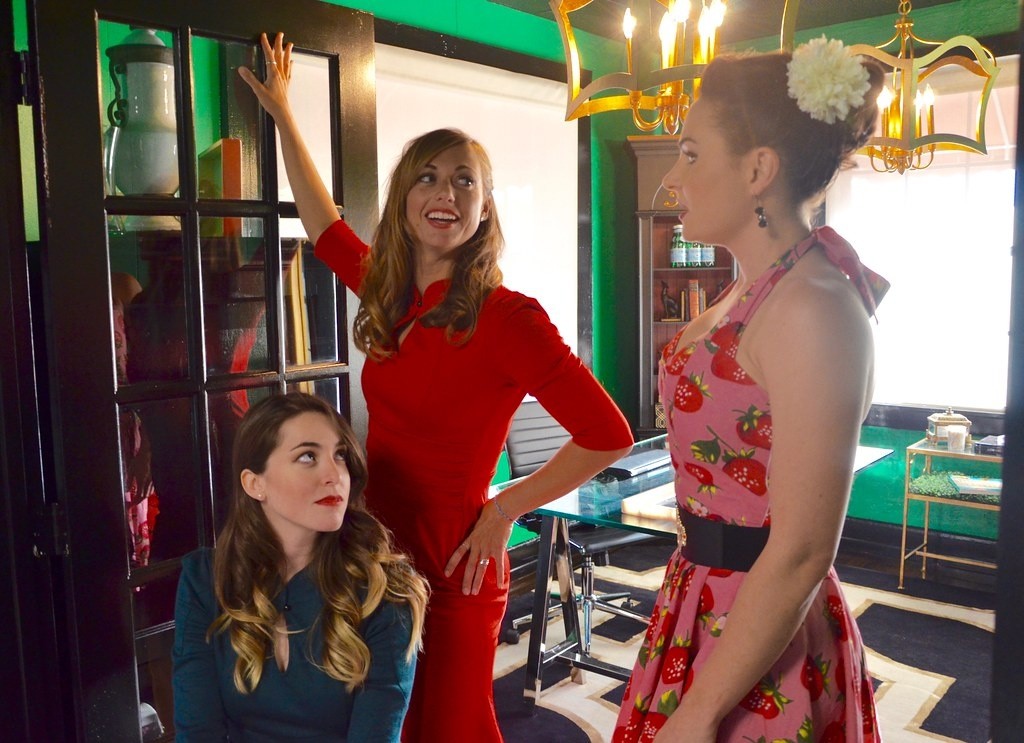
[669,224,715,268]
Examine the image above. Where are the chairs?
[506,401,670,657]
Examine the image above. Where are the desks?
[493,434,895,715]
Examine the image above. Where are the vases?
[98,24,183,233]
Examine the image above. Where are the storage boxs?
[925,406,973,446]
[198,137,243,238]
[975,434,1005,457]
[948,475,1003,495]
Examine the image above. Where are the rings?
[480,559,489,564]
[463,542,468,550]
[265,60,276,66]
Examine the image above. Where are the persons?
[174,392,432,743]
[109,181,306,597]
[611,37,886,743]
[237,32,634,743]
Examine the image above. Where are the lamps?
[550,0,801,136]
[847,0,1003,176]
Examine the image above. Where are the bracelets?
[493,494,516,523]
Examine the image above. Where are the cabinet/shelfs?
[628,136,739,445]
[898,438,1003,592]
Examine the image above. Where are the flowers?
[784,33,872,126]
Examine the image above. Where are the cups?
[946,424,966,453]
